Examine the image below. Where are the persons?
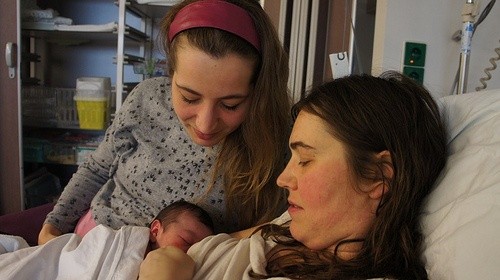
[145,199,219,259]
[38,0,295,244]
[137,70,450,280]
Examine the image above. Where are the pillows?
[417,88,500,279]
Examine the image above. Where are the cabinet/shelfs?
[20,0,152,193]
